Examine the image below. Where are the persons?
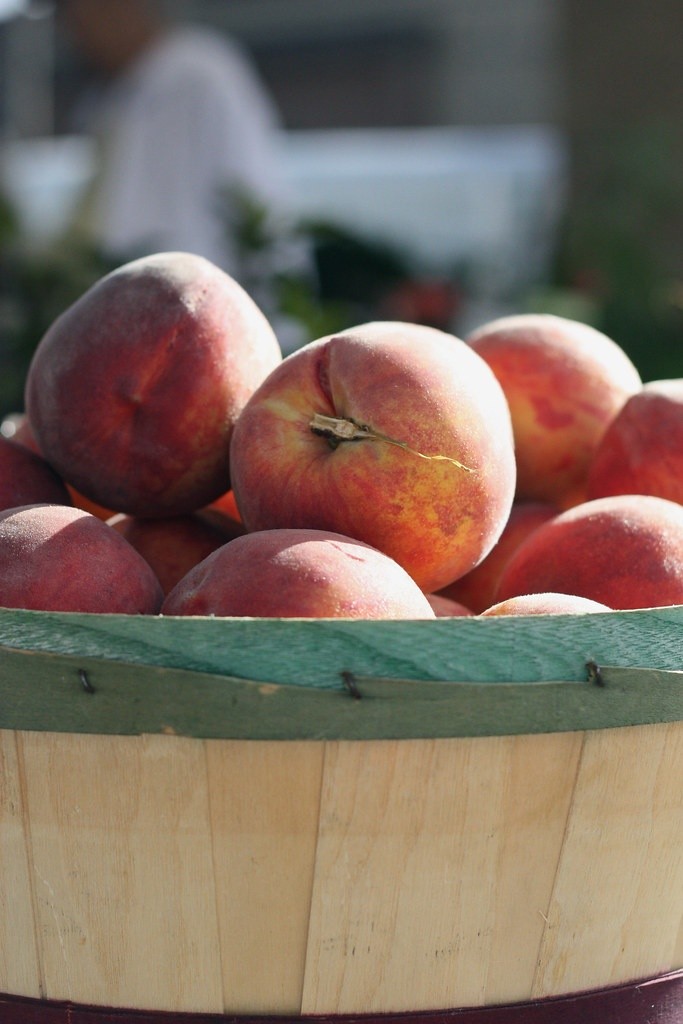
[65,0,279,269]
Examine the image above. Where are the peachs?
[0,249,683,619]
